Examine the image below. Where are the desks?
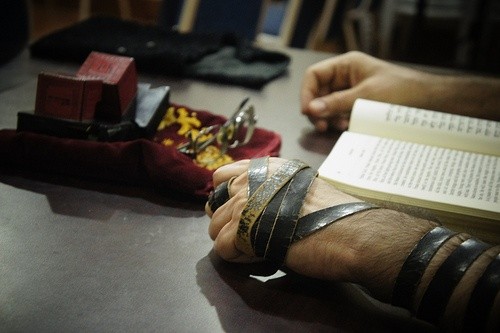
[0,41,499,331]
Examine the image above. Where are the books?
[316,97,500,229]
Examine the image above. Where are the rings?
[208,175,238,215]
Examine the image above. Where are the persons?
[203,47,500,333]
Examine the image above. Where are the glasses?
[179,98,256,163]
